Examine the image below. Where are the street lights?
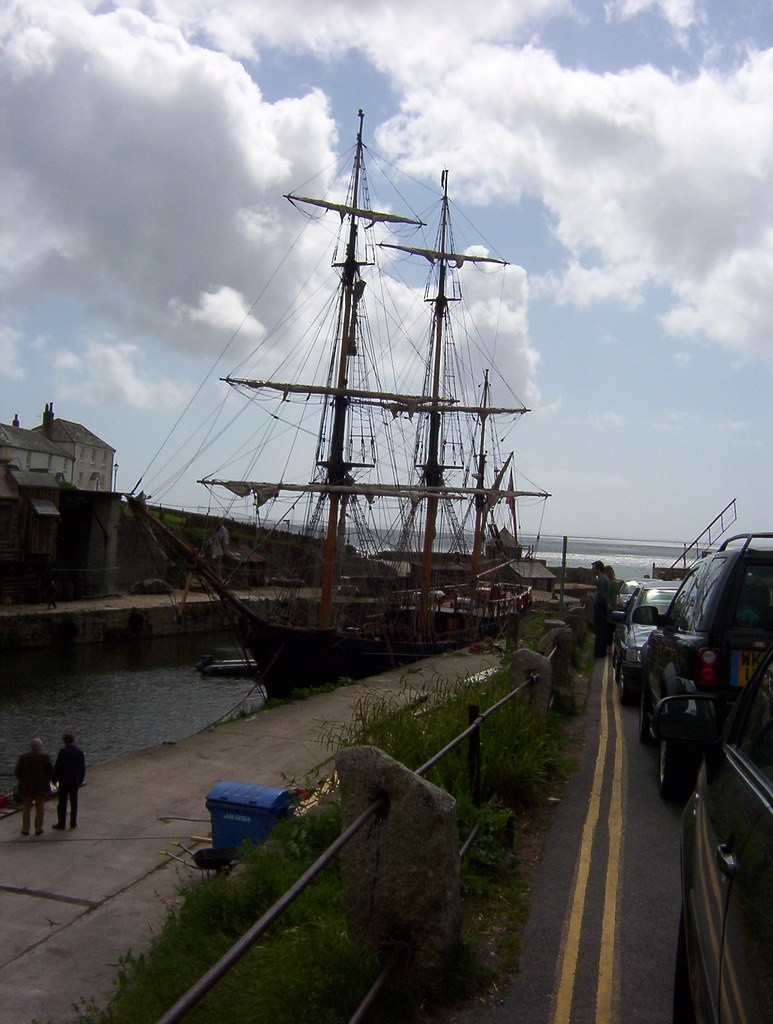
[113,461,119,492]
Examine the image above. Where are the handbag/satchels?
[618,601,624,609]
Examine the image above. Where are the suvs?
[654,645,773,1024]
[630,530,773,801]
[616,580,640,608]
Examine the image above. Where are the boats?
[194,654,259,676]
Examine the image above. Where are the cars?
[610,579,685,706]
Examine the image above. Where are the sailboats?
[121,106,555,702]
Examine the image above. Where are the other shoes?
[70,824,77,829]
[35,830,44,835]
[21,830,29,835]
[52,824,65,830]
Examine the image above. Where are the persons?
[603,565,623,655]
[592,560,613,659]
[14,738,52,836]
[51,732,85,830]
